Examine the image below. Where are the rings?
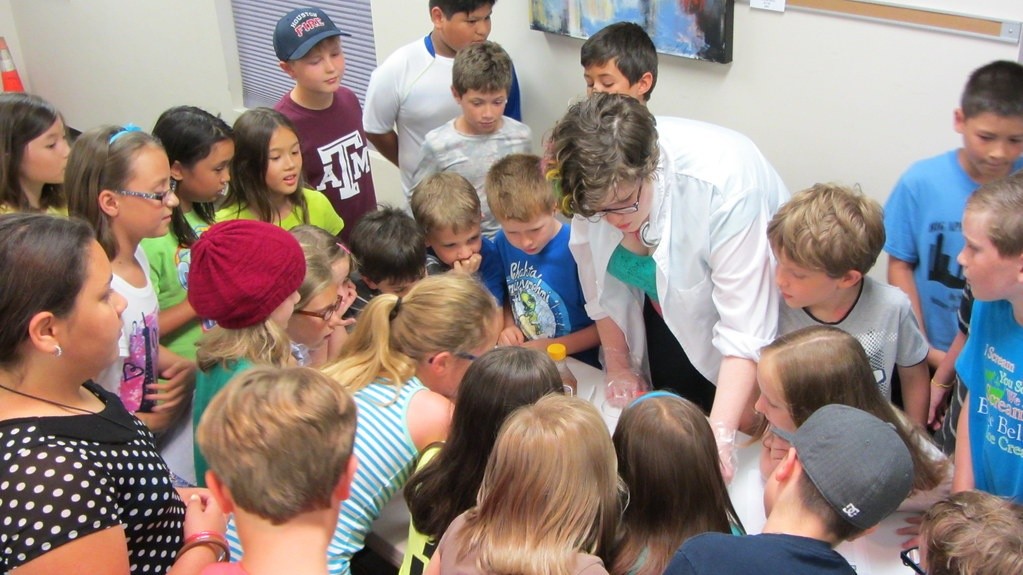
[615,393,631,398]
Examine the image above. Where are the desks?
[365,355,920,575]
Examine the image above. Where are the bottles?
[547,343,577,398]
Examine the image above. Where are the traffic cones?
[0,38,25,94]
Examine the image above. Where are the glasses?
[293,295,345,321]
[591,177,644,217]
[900,545,925,575]
[117,180,178,206]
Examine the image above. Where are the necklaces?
[0,383,177,484]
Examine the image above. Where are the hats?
[186,218,306,328]
[773,404,915,529]
[273,7,351,62]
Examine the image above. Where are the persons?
[0,0,1023,575]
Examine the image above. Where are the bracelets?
[932,379,956,387]
[176,531,231,561]
